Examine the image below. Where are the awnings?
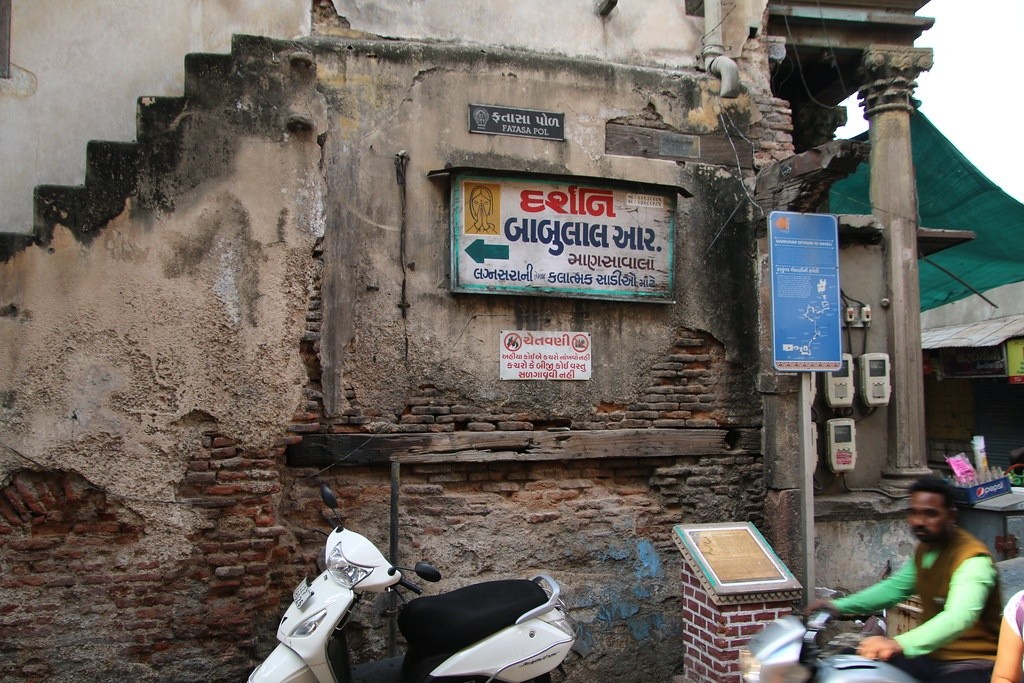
[809,98,1024,313]
[920,313,1024,350]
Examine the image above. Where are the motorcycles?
[738,609,925,683]
[247,483,576,683]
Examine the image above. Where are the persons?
[803,475,1004,683]
[991,589,1024,683]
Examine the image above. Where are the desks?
[957,505,1024,559]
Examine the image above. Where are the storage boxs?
[949,475,1011,505]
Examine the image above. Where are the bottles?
[954,466,1004,487]
